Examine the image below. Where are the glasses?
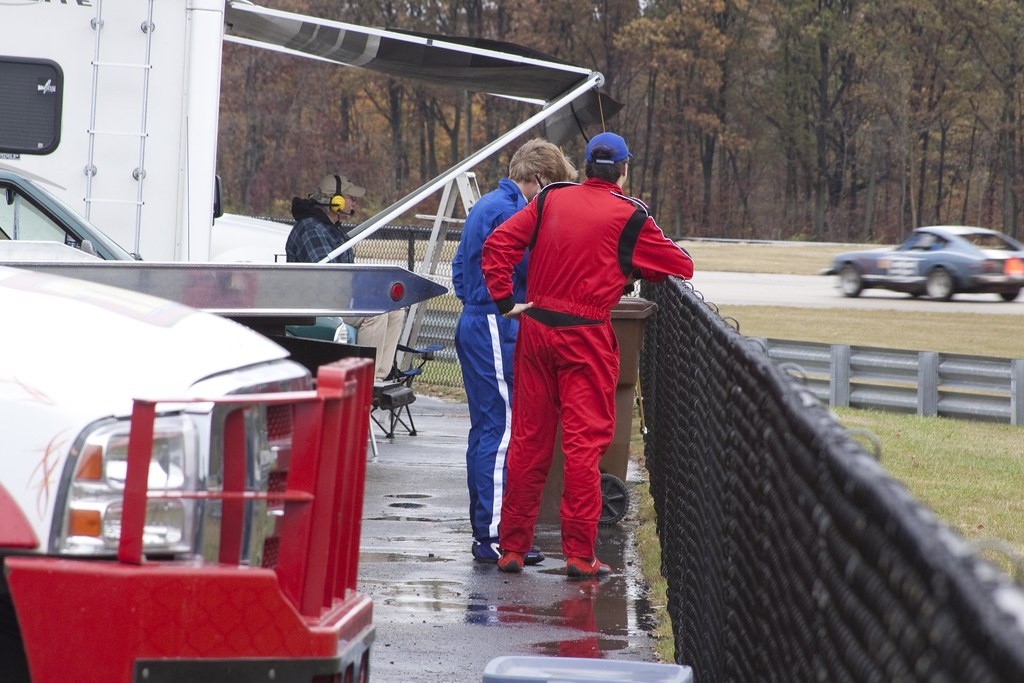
[341,196,357,201]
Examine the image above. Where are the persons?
[478,131,696,576]
[283,171,411,387]
[451,137,578,567]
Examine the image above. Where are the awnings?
[222,0,623,147]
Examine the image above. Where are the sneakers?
[472,538,546,564]
[497,549,525,572]
[566,555,613,579]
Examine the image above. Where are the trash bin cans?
[535,297,659,526]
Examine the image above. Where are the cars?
[0,162,140,259]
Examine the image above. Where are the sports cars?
[819,225,1024,301]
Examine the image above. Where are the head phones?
[330,174,344,213]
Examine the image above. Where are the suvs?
[0,263,380,683]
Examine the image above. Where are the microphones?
[337,208,355,216]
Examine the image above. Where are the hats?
[318,174,367,199]
[586,132,634,165]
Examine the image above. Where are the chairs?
[370,344,446,440]
